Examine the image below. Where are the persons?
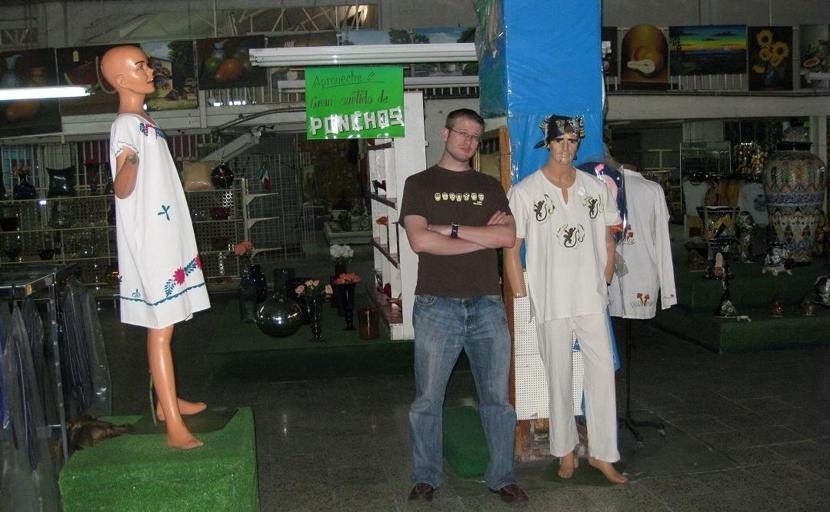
[100,44,212,451]
[502,112,675,484]
[400,108,530,505]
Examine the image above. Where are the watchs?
[450,221,460,239]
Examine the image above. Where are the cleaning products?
[238,263,267,324]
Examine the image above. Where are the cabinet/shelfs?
[0,177,283,310]
[367,91,426,341]
[650,278,830,354]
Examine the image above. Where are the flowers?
[330,243,354,265]
[334,271,362,286]
[295,279,333,303]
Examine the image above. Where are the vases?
[335,257,345,278]
[696,141,830,320]
[339,284,356,330]
[304,298,327,344]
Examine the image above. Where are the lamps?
[247,43,478,67]
[276,75,480,93]
[0,85,94,101]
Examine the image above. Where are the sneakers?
[408,483,434,504]
[488,483,528,502]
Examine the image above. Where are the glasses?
[447,126,479,140]
